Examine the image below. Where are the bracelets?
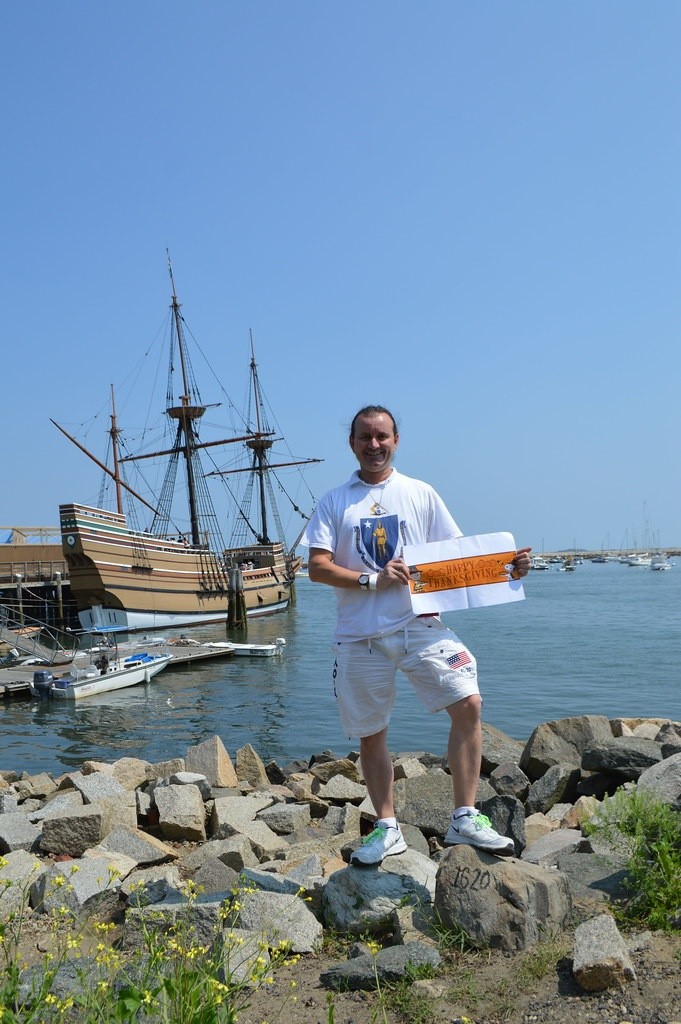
[370,573,379,591]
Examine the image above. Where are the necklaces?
[363,485,383,515]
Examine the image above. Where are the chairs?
[67,663,101,683]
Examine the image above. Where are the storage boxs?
[53,678,72,689]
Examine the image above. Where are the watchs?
[359,573,370,591]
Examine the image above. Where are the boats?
[531,557,550,570]
[86,636,166,651]
[27,651,173,700]
[202,638,287,656]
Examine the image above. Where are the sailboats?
[544,500,671,571]
[49,243,326,635]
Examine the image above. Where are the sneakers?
[348,822,409,868]
[444,810,515,858]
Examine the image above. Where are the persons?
[302,405,532,864]
